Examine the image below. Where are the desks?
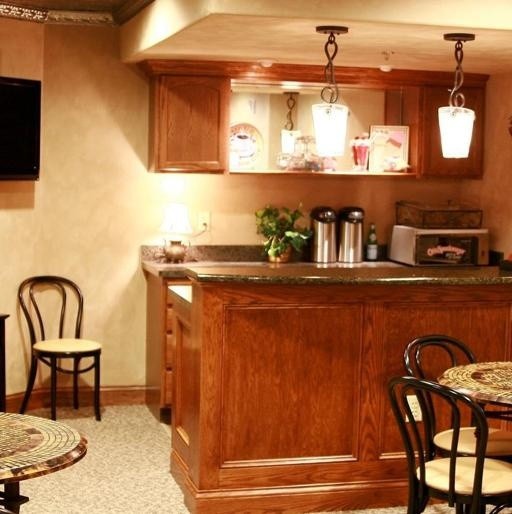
[0,409,87,514]
[437,360,512,406]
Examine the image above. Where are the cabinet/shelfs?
[148,75,230,173]
[145,276,192,423]
[401,86,485,181]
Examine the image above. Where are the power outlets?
[197,211,210,232]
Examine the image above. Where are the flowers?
[255,200,311,257]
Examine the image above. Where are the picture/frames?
[368,124,409,173]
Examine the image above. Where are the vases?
[267,243,293,262]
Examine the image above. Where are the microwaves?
[387,225,490,268]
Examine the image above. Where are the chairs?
[17,275,102,421]
[402,334,512,458]
[389,375,512,514]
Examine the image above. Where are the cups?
[351,146,368,172]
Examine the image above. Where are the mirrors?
[229,78,385,173]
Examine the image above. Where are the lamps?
[160,204,191,264]
[281,91,299,154]
[437,32,476,159]
[311,25,350,156]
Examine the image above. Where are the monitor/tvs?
[0,76,42,181]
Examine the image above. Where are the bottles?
[366,222,379,262]
